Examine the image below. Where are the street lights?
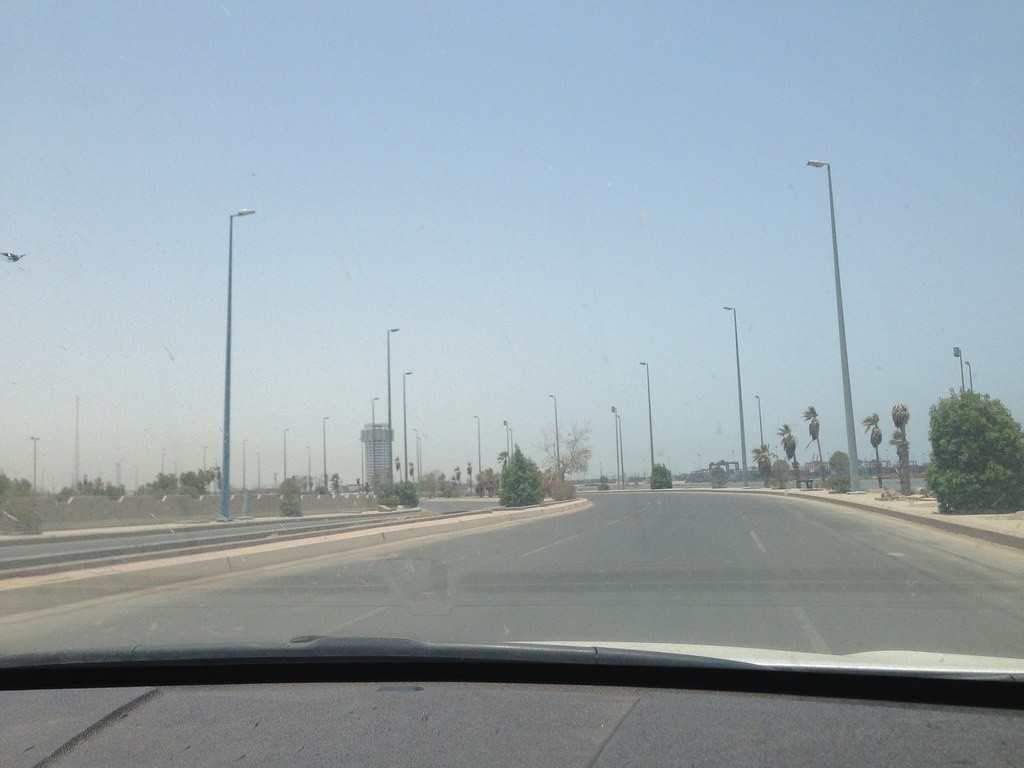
[549,394,561,473]
[412,429,423,479]
[243,439,248,489]
[472,415,482,472]
[611,406,625,491]
[388,328,399,487]
[403,371,413,481]
[755,395,764,448]
[31,435,40,492]
[807,159,866,496]
[322,416,330,486]
[723,306,751,489]
[216,207,255,521]
[965,361,974,393]
[73,394,80,485]
[640,361,655,467]
[953,346,965,391]
[256,452,261,491]
[282,427,290,480]
[371,396,380,484]
[503,420,509,459]
[509,427,513,457]
